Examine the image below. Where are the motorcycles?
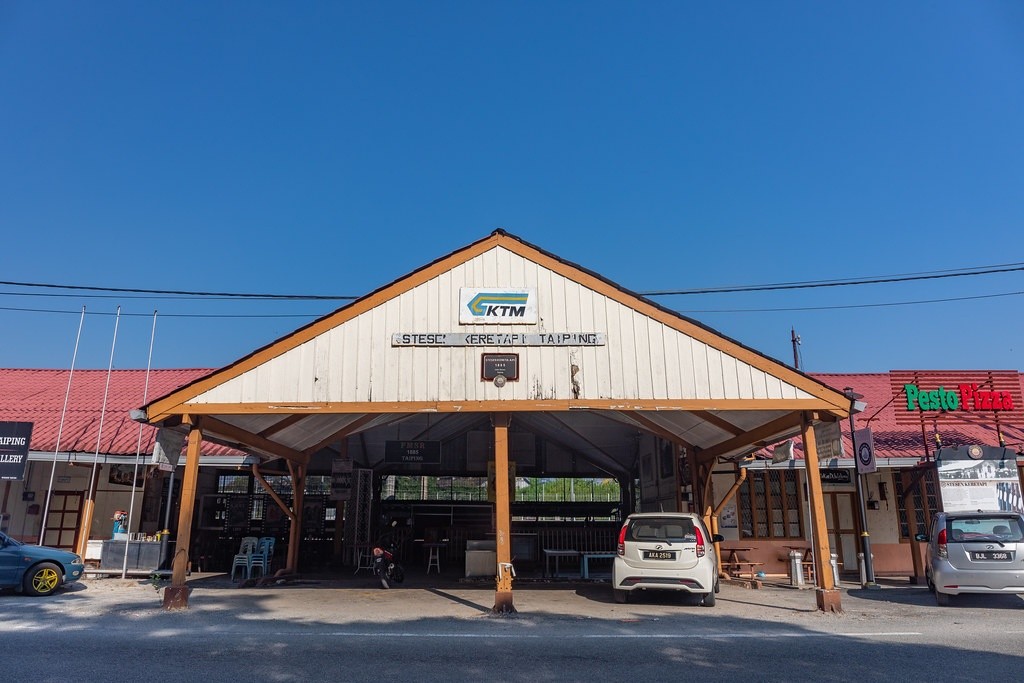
[370,544,408,590]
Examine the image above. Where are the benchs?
[722,560,750,577]
[720,562,765,580]
[801,561,815,581]
[778,555,813,578]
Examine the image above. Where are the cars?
[0,530,85,597]
[915,509,1024,606]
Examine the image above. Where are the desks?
[719,547,758,578]
[346,543,384,576]
[100,540,176,572]
[781,546,812,580]
[580,551,617,579]
[423,543,446,575]
[543,549,581,580]
[464,540,497,578]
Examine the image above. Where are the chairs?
[992,525,1013,539]
[952,528,963,540]
[230,536,275,582]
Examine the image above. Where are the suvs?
[611,510,725,606]
